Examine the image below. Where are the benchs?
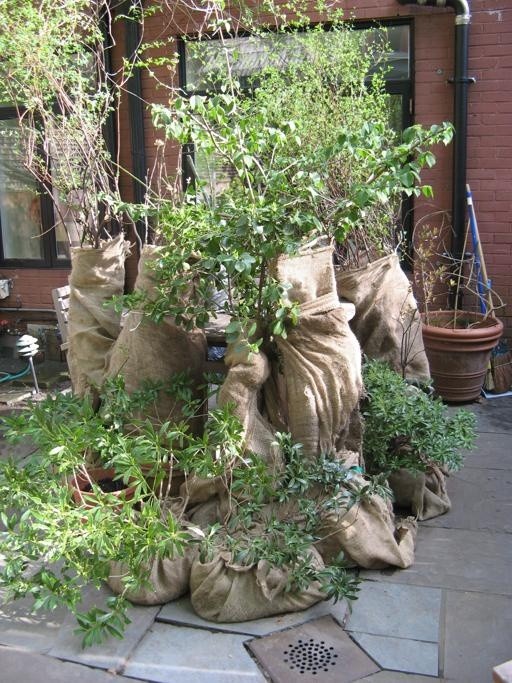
[51,283,73,383]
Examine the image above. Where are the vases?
[140,460,188,498]
[67,465,144,523]
[416,308,503,403]
